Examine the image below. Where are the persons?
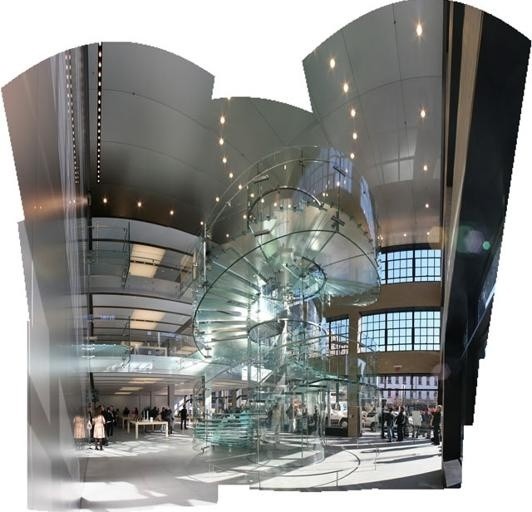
[266,402,324,438]
[177,405,188,430]
[378,403,443,447]
[86,403,174,452]
[73,408,85,450]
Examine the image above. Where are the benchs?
[324,426,347,436]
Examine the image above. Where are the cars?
[362,412,378,432]
[324,401,347,428]
[384,412,413,433]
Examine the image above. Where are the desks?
[134,345,167,356]
[118,415,169,440]
